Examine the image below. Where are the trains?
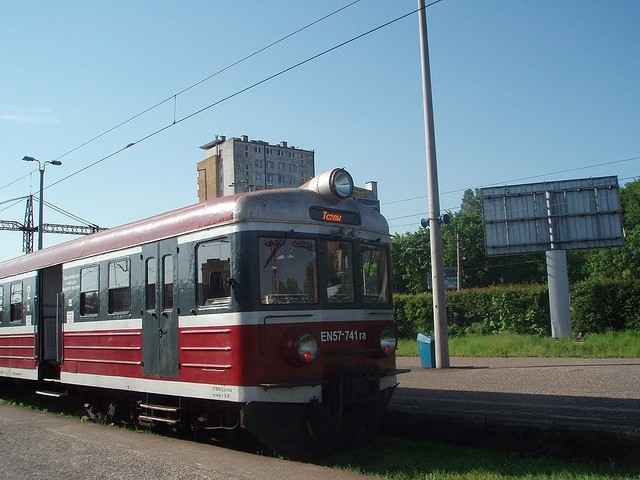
[0,168,412,452]
